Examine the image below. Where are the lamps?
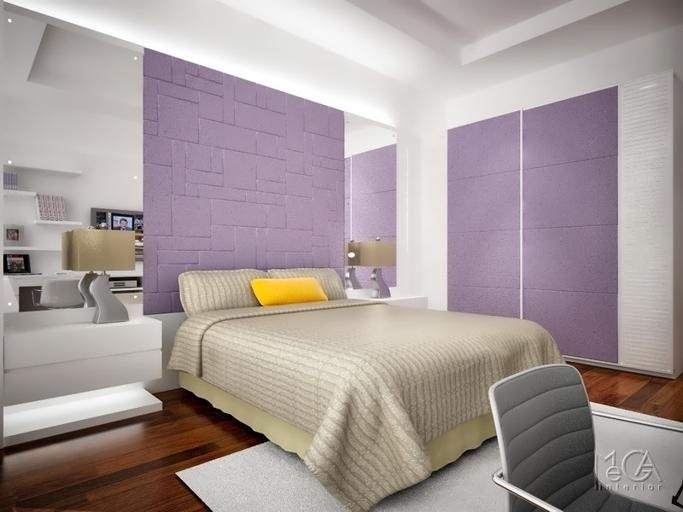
[360,237,396,298]
[62,231,98,309]
[345,239,362,289]
[72,223,135,324]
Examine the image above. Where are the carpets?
[175,401,683,512]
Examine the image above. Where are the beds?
[167,299,566,512]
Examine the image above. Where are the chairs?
[488,363,683,512]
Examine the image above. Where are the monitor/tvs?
[111,212,134,232]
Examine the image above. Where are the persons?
[134,217,143,228]
[113,219,132,231]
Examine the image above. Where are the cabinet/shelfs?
[0,304,162,448]
[93,270,144,304]
[0,162,82,313]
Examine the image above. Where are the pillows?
[178,268,348,318]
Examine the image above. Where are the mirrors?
[344,111,397,288]
[0,1,144,313]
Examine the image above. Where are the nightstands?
[361,296,428,308]
